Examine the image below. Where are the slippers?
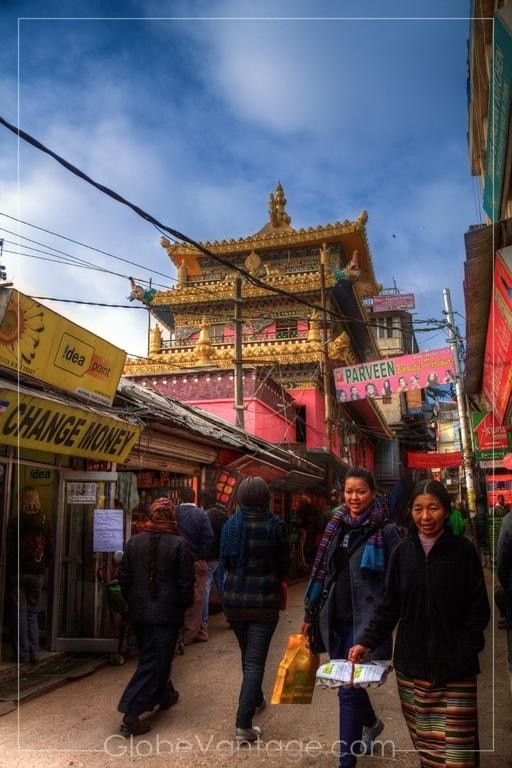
[162,691,178,709]
[120,723,162,736]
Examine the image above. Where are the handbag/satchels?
[308,614,326,653]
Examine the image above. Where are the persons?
[217,475,290,742]
[343,479,491,768]
[117,498,191,734]
[175,489,214,650]
[285,494,334,577]
[300,467,401,768]
[198,492,230,645]
[450,490,511,675]
[6,488,49,664]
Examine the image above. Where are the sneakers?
[236,727,262,740]
[255,699,266,713]
[360,721,384,754]
